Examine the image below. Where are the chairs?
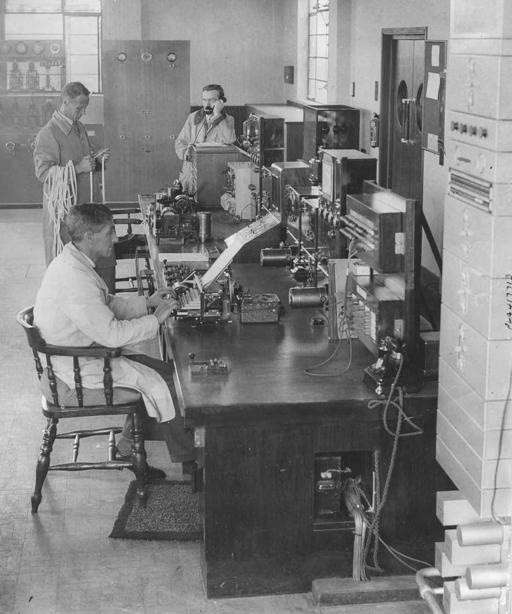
[99,208,155,295]
[17,304,153,516]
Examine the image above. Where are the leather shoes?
[115,450,166,482]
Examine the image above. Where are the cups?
[198,211,211,239]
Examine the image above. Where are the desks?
[139,192,431,596]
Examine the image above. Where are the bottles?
[9,61,38,91]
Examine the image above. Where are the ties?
[73,121,81,136]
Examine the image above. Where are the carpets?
[108,477,203,541]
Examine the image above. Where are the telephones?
[362,336,413,394]
[205,96,227,115]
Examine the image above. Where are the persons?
[35,82,111,264]
[174,84,238,188]
[34,202,200,481]
